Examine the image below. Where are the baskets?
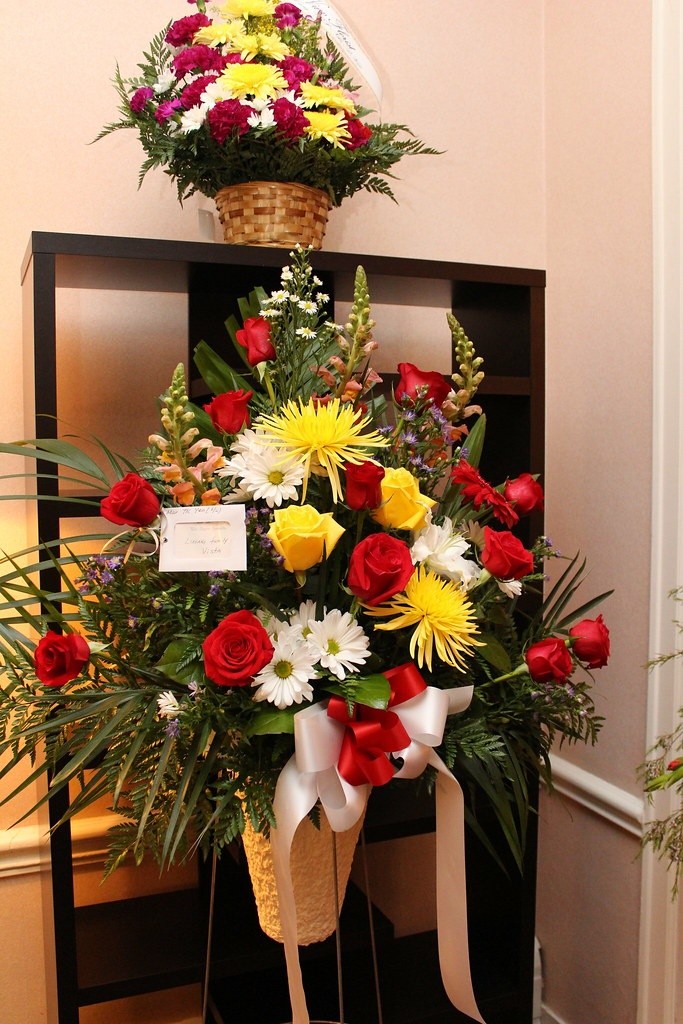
[214,181,332,251]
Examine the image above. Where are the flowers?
[86,0,447,211]
[0,238,618,888]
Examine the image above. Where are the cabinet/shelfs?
[18,232,545,1024]
